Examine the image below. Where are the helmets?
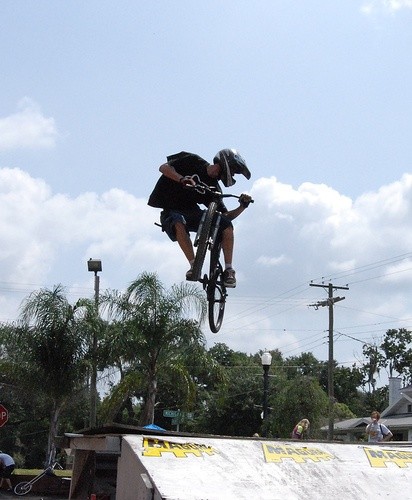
[213,147,251,187]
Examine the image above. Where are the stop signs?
[0,404,9,427]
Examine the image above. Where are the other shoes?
[186,264,195,276]
[223,268,236,288]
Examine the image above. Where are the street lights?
[261,352,273,438]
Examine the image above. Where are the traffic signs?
[164,409,192,425]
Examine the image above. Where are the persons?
[292,418,310,440]
[365,411,394,442]
[0,452,15,492]
[146,147,252,289]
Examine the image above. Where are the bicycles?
[13,461,63,496]
[186,183,254,333]
